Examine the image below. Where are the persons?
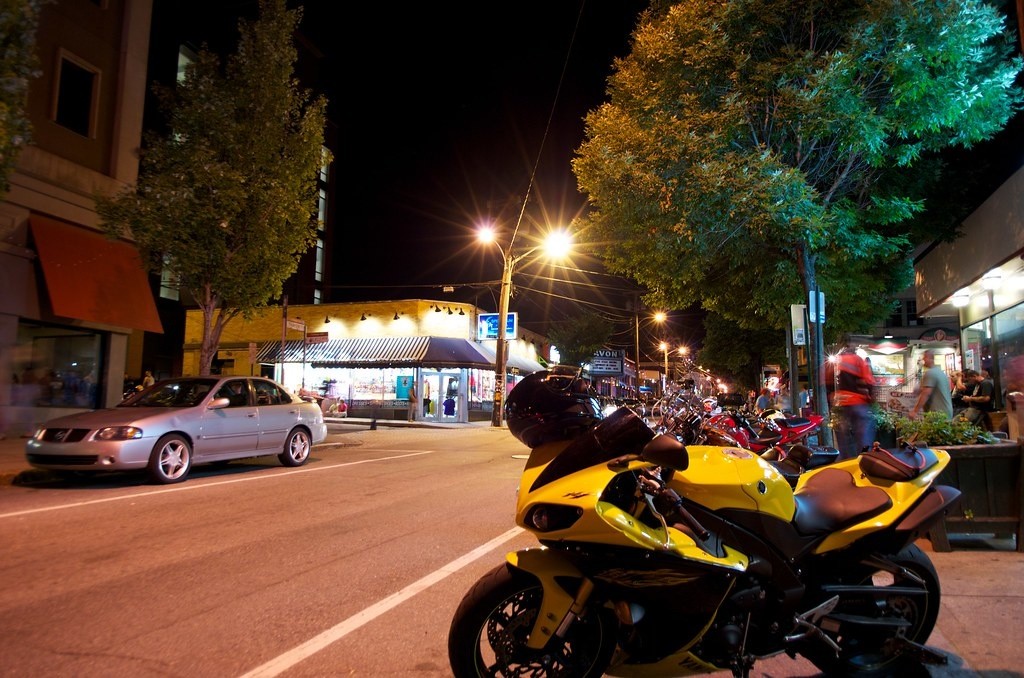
[799,382,810,408]
[124,372,133,384]
[38,371,65,405]
[982,369,994,384]
[738,405,744,415]
[216,381,247,406]
[907,350,954,422]
[325,399,347,417]
[951,369,970,401]
[141,370,154,389]
[756,388,770,414]
[824,333,877,460]
[408,388,418,422]
[744,402,754,414]
[953,369,996,422]
[685,386,692,393]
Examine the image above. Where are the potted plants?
[832,403,1024,552]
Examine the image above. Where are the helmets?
[504,371,604,448]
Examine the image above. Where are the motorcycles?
[444,365,962,678]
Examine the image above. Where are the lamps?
[325,312,336,323]
[360,312,371,320]
[430,302,441,312]
[521,334,526,341]
[884,329,893,338]
[394,308,404,319]
[455,307,465,315]
[443,305,453,315]
[530,338,534,344]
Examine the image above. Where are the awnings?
[27,213,164,333]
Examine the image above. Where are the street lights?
[635,310,667,398]
[475,225,572,427]
[658,341,689,377]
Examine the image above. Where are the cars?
[24,374,329,485]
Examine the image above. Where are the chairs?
[225,385,241,406]
[975,395,996,428]
